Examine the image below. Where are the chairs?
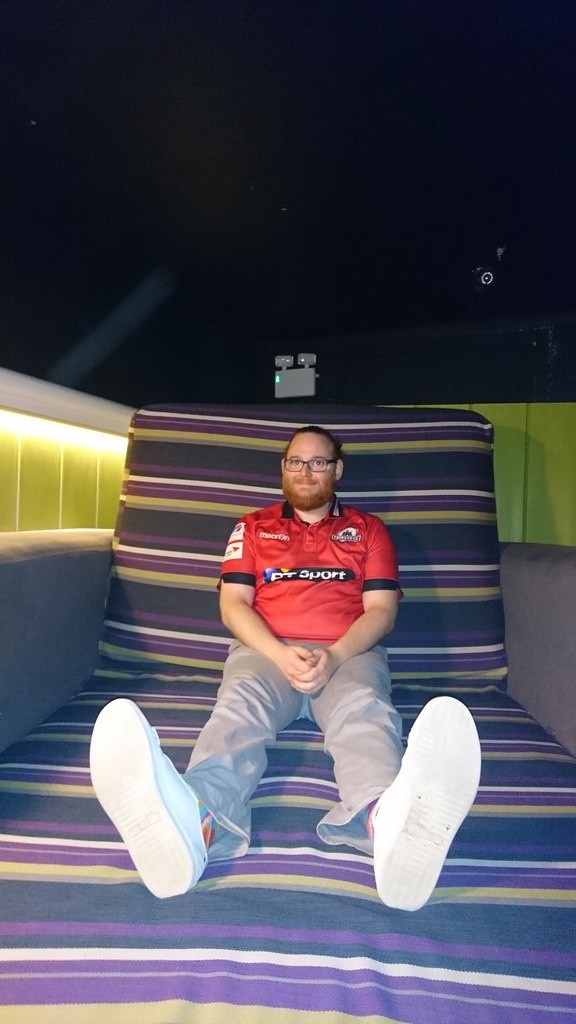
[0,405,576,1024]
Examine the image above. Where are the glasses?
[284,458,337,472]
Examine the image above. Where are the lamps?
[274,352,320,398]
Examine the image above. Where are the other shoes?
[372,696,481,912]
[89,698,208,899]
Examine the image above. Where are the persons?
[87,426,482,912]
